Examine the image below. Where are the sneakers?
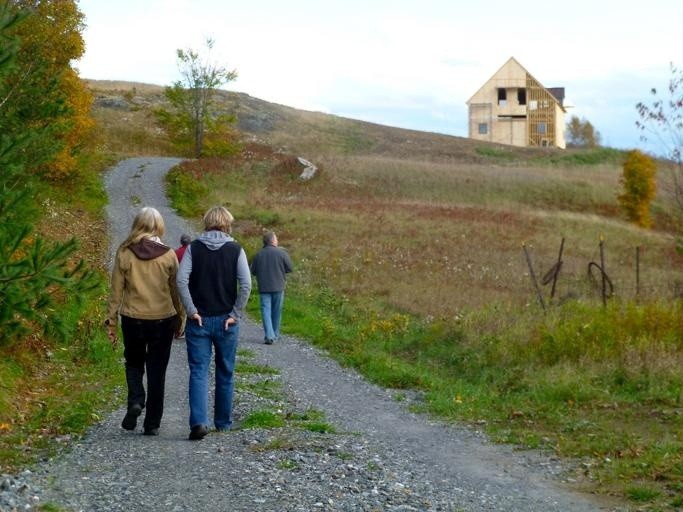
[122,403,142,430]
[265,338,274,344]
[144,424,159,435]
[189,424,209,439]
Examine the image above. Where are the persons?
[175,234,192,339]
[250,231,293,344]
[176,206,252,441]
[103,207,188,436]
